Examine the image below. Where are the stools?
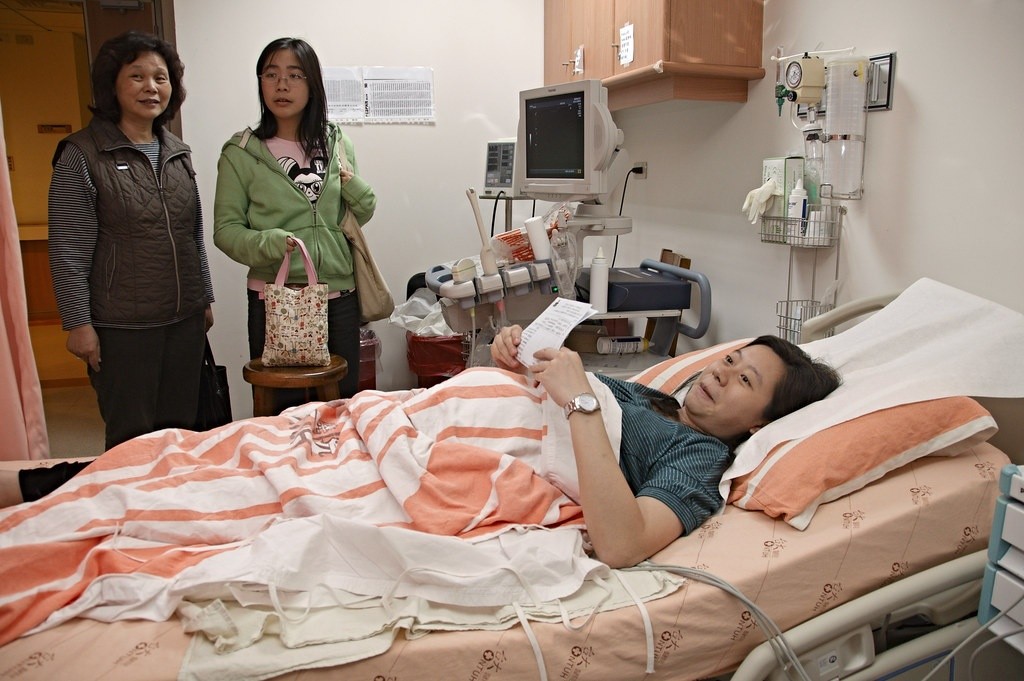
[242,353,349,418]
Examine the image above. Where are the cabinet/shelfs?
[543,0,765,112]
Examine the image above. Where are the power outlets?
[632,161,647,179]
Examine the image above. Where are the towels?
[711,276,1024,514]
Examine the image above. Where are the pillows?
[623,337,995,529]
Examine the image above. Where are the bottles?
[787,178,808,244]
[589,247,608,313]
[597,336,655,354]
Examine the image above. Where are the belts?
[246,278,356,300]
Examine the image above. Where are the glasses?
[258,72,309,88]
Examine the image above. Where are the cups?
[805,211,829,245]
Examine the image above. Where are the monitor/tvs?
[519,79,608,194]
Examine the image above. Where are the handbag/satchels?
[194,332,233,432]
[262,237,331,367]
[335,139,394,323]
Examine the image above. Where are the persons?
[45,29,215,453]
[211,38,379,418]
[0,324,843,566]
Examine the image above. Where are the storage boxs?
[761,156,805,240]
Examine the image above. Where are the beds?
[1,278,1024,681]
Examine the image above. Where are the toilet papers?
[524,216,554,261]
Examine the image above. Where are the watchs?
[564,390,602,420]
